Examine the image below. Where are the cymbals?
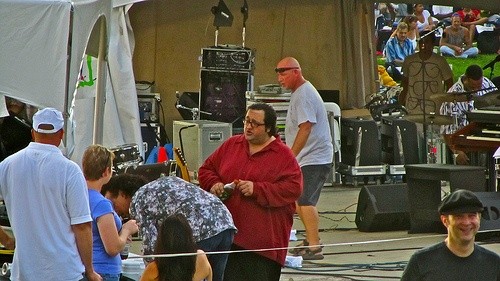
[441,92,474,102]
[474,90,500,111]
[406,114,462,125]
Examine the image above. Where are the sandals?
[289,239,324,254]
[294,249,324,260]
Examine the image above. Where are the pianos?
[448,121,500,152]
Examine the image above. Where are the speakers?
[355,183,410,232]
[198,69,254,130]
[471,192,500,242]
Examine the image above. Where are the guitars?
[174,148,191,183]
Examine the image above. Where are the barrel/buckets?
[376,30,393,52]
[477,24,499,54]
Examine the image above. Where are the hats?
[33,108,64,134]
[438,189,484,214]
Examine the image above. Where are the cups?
[395,66,402,73]
[483,22,494,27]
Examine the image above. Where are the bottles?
[433,44,440,55]
[120,234,129,260]
[216,179,240,202]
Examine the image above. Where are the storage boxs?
[141,123,161,163]
[335,162,386,175]
[386,164,407,174]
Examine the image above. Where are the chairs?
[324,101,342,184]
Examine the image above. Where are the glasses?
[275,68,299,73]
[243,119,265,127]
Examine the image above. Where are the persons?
[103,174,238,281]
[373,2,500,76]
[139,213,212,281]
[198,103,302,281]
[82,144,139,281]
[275,57,334,260]
[399,30,454,115]
[440,64,498,166]
[0,107,102,281]
[399,189,500,281]
